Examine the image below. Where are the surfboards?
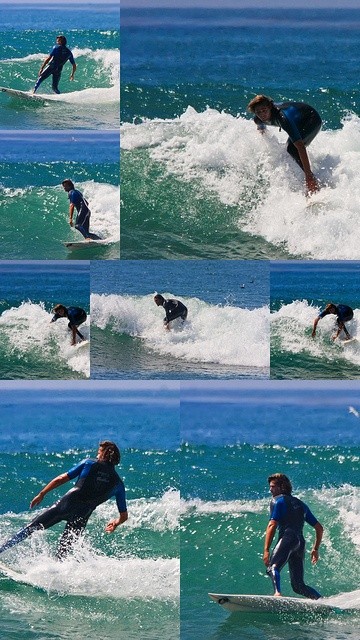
[0,87,41,99]
[64,237,107,246]
[210,593,359,612]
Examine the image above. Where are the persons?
[50,302,87,346]
[0,439,130,565]
[310,299,355,344]
[249,94,323,197]
[31,34,77,96]
[59,179,103,241]
[263,471,324,602]
[151,294,189,333]
